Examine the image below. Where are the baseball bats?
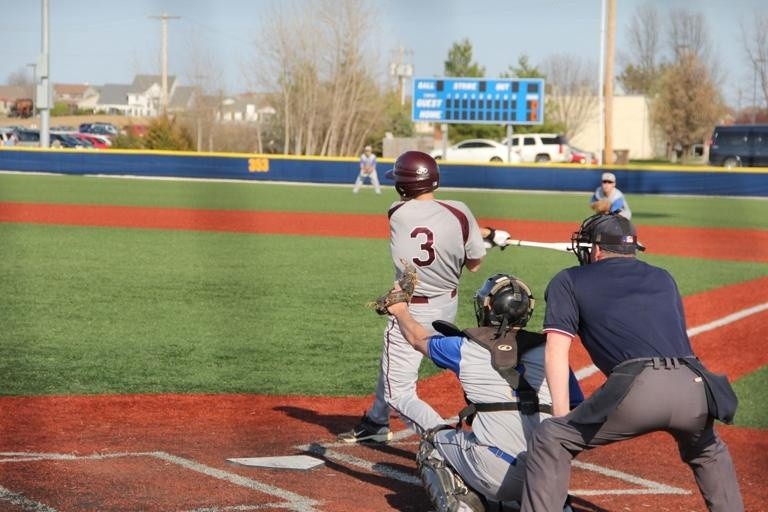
[485,239,592,255]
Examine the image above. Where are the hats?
[601,173,616,183]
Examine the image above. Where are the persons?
[590,172,631,219]
[352,144,380,194]
[336,150,510,443]
[519,211,745,512]
[384,273,584,511]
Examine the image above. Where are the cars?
[0,122,152,150]
[429,138,522,164]
[568,145,598,165]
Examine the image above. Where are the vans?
[709,125,768,168]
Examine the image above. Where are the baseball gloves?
[373,257,417,315]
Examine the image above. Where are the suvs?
[499,132,573,165]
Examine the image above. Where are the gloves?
[483,226,511,251]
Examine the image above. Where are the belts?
[411,286,457,304]
[643,359,687,369]
[487,445,519,467]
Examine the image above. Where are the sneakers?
[337,418,392,443]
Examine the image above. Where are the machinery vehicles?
[9,99,34,119]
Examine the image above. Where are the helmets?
[383,151,440,200]
[570,212,638,266]
[474,274,534,328]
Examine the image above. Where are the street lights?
[26,63,37,130]
